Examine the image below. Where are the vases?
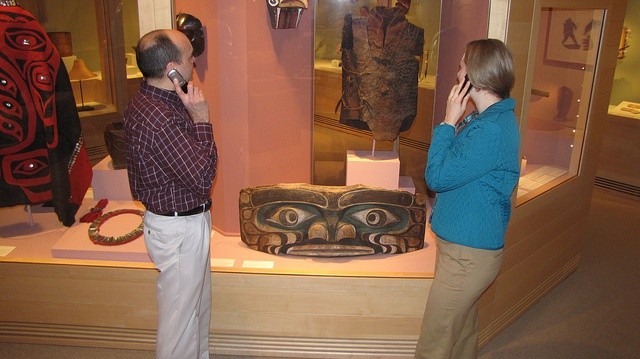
[554,86,574,122]
[104,120,127,170]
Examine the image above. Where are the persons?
[414,39,522,359]
[123,28,218,359]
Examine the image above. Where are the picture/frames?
[542,6,605,70]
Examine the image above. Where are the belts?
[160,200,212,216]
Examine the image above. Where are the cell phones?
[458,73,473,97]
[167,69,188,94]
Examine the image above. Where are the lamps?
[68,58,98,112]
